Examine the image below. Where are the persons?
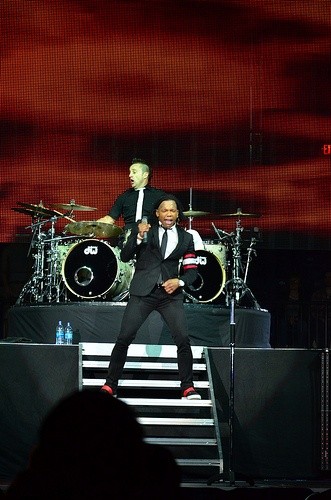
[97,158,188,249]
[2,389,179,500]
[101,195,202,400]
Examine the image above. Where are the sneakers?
[101,383,117,397]
[181,387,201,400]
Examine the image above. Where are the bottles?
[65,322,73,344]
[55,320,64,344]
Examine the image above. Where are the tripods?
[14,207,76,306]
[218,216,263,309]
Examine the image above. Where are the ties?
[134,189,144,223]
[161,229,168,259]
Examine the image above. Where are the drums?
[47,241,230,304]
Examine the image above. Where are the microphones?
[142,215,149,244]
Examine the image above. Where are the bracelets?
[137,233,143,239]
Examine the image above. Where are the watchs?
[179,280,184,286]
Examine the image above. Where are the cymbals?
[11,201,63,219]
[220,211,256,216]
[47,203,98,212]
[182,211,211,216]
[64,220,123,238]
